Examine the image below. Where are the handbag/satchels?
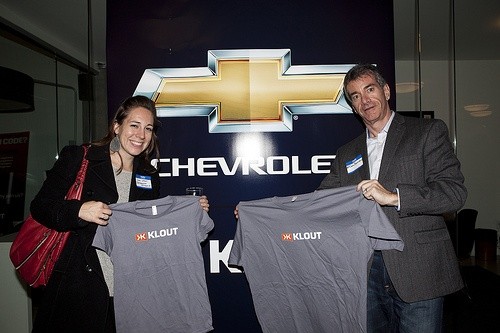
[9,145,88,289]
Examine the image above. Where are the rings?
[101,213,105,220]
[364,188,368,192]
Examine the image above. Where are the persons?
[26,96,209,333]
[234,63,469,333]
